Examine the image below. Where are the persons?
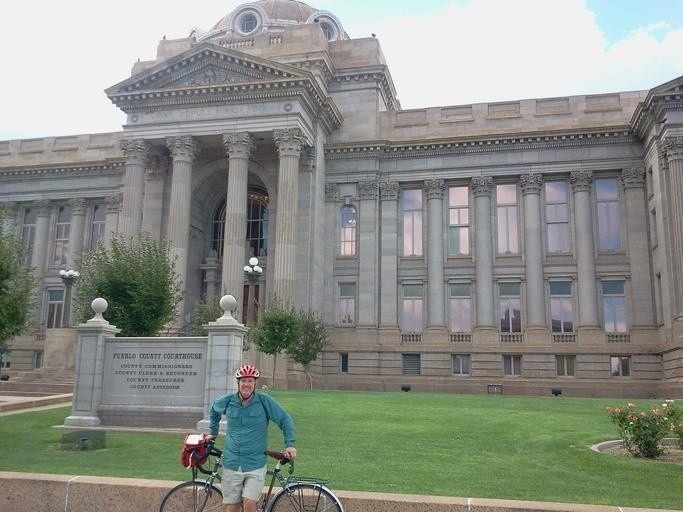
[202,364,297,512]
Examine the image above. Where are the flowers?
[600,395,680,455]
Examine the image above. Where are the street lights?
[244,257,263,327]
[59,269,80,328]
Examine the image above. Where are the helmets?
[235,364,260,378]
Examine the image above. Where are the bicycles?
[159,439,342,512]
